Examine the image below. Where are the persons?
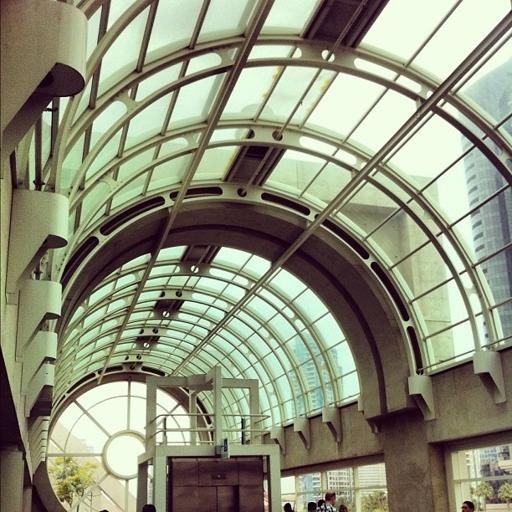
[308,502,316,512]
[339,504,348,512]
[284,503,294,512]
[316,491,337,512]
[461,500,474,512]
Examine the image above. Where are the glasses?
[461,506,467,509]
